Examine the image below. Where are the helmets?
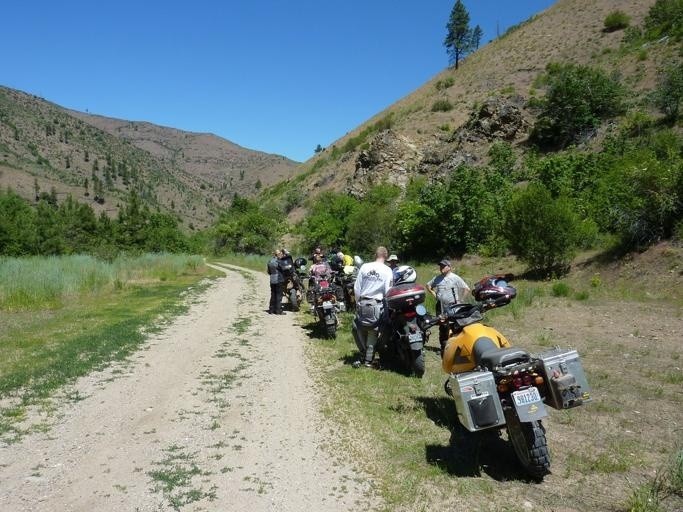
[342,255,353,265]
[474,273,517,301]
[313,255,321,263]
[391,265,416,286]
[294,258,306,267]
[336,252,343,260]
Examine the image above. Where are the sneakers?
[268,311,286,315]
[364,360,372,366]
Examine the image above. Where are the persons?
[442,321,511,478]
[267,244,470,368]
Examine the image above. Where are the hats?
[385,255,399,263]
[280,249,290,255]
[438,260,450,266]
[273,250,280,254]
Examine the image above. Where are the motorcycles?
[279,258,362,338]
[414,300,593,474]
[376,265,431,378]
